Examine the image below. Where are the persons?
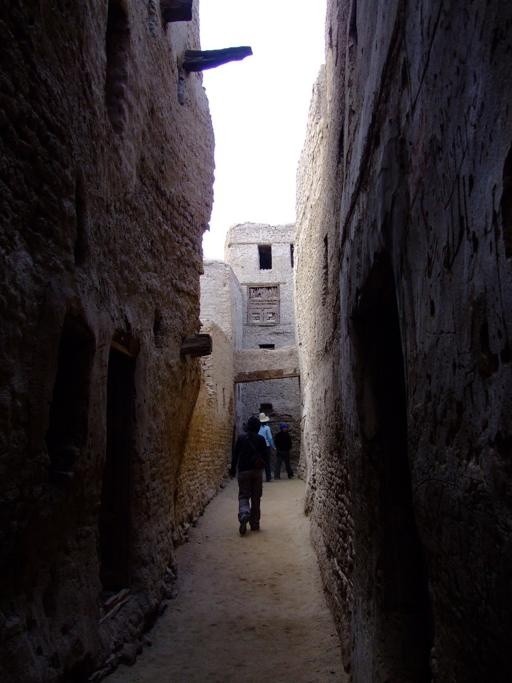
[256,412,276,483]
[272,422,298,480]
[228,414,267,536]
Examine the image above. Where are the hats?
[280,423,288,428]
[259,412,270,422]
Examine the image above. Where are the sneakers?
[239,514,260,536]
